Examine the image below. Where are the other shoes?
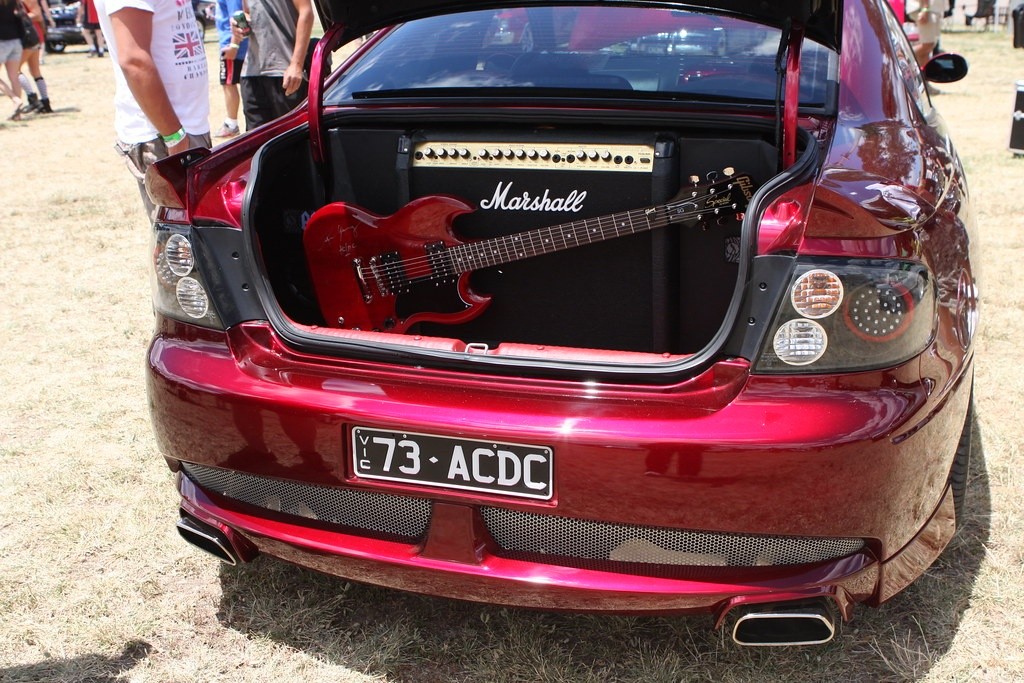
[211,122,242,138]
[8,112,21,120]
[98,52,103,57]
[33,98,52,113]
[20,93,39,113]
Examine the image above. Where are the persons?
[0,0,57,122]
[906,0,949,96]
[92,0,213,226]
[76,0,104,58]
[213,0,314,140]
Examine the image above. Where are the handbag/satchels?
[14,2,39,49]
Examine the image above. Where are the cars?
[39,0,111,54]
[142,0,978,647]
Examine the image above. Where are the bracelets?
[48,17,53,20]
[162,127,187,148]
[919,7,929,13]
